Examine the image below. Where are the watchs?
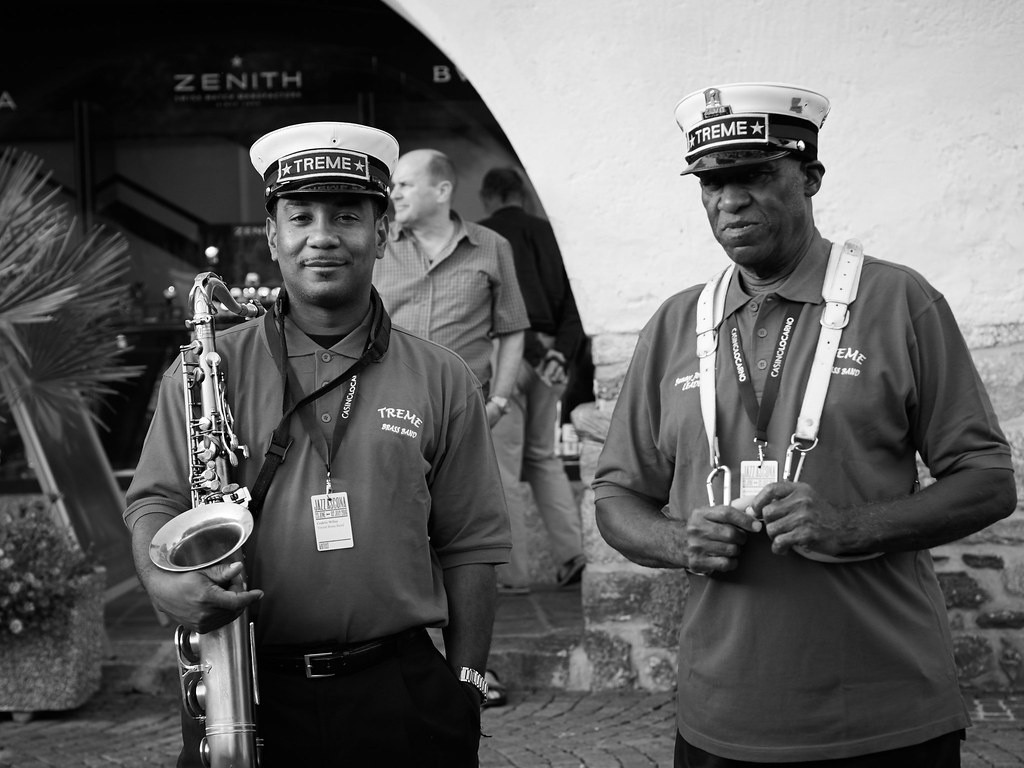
[456,666,489,707]
[490,395,512,415]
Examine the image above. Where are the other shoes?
[557,554,587,587]
[496,580,531,595]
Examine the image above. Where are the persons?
[475,167,587,594]
[591,84,1017,768]
[371,149,530,707]
[122,122,513,768]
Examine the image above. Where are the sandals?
[481,666,507,708]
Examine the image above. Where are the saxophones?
[146,268,271,768]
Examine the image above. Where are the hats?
[249,121,400,219]
[675,83,828,175]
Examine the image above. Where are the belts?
[257,624,422,679]
